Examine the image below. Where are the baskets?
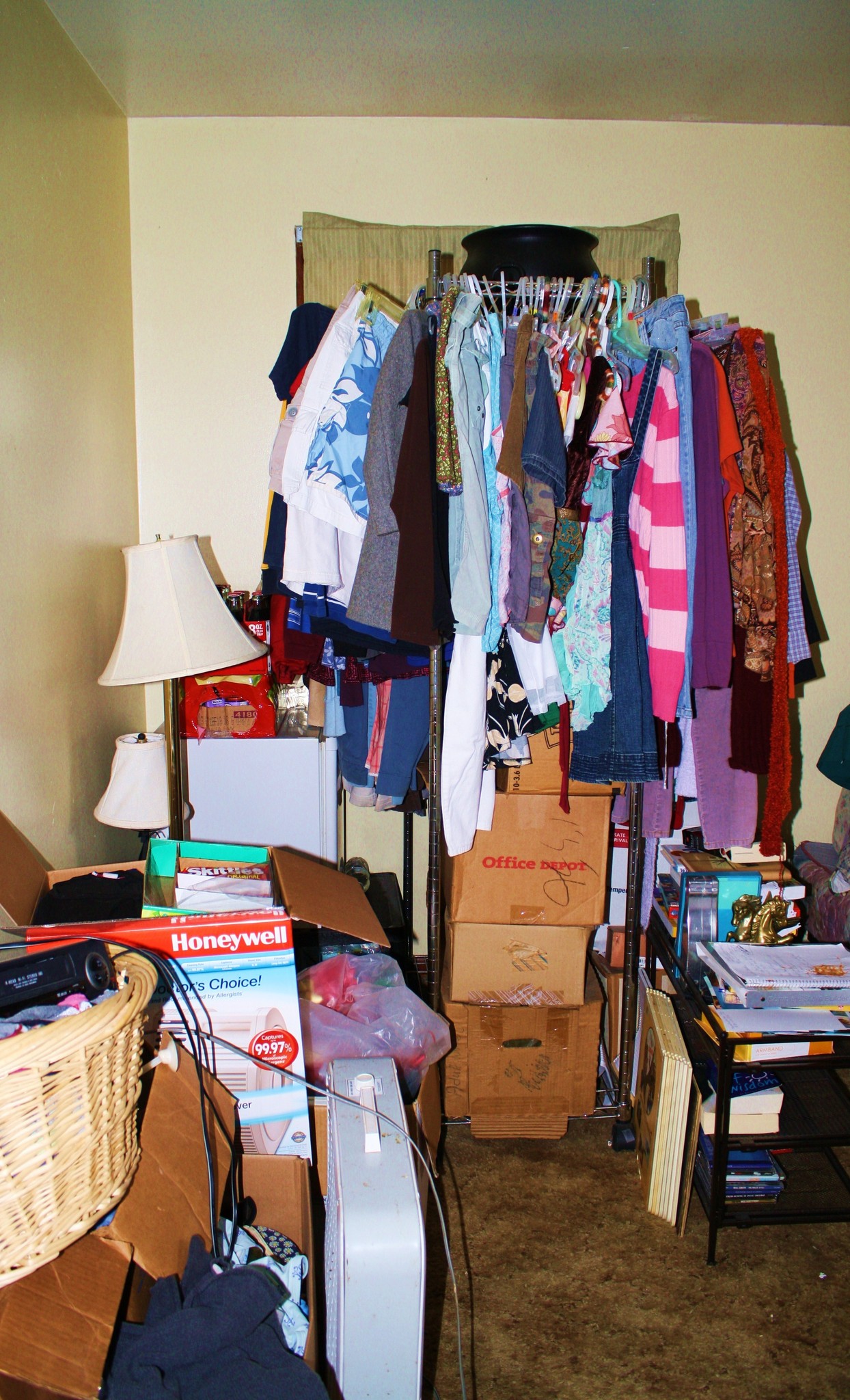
[0,943,158,1288]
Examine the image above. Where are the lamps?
[93,731,194,860]
[97,533,270,841]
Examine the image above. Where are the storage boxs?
[0,717,625,1400]
[179,619,275,739]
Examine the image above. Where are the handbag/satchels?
[296,953,451,1092]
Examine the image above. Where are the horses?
[724,895,800,945]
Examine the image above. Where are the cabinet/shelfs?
[643,870,849,1264]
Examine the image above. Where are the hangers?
[344,272,667,400]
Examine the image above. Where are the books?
[690,1052,783,1135]
[711,940,849,988]
[695,1131,786,1205]
[652,825,806,940]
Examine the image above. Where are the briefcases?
[323,1055,425,1399]
